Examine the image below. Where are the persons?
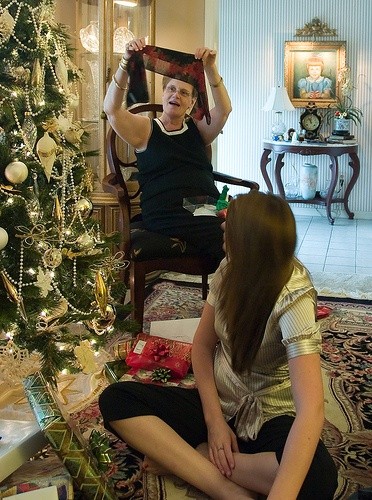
[98,190,338,500]
[103,37,233,286]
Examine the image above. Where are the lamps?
[263,86,295,142]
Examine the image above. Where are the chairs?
[102,102,260,338]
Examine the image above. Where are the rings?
[218,448,224,450]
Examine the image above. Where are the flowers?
[323,95,363,127]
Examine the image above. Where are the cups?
[297,130,306,141]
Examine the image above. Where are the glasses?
[166,85,193,97]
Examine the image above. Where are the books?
[328,130,356,143]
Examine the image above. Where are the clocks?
[299,102,321,140]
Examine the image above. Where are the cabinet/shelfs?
[76,0,156,287]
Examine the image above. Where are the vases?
[333,119,350,131]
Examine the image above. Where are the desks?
[260,141,361,226]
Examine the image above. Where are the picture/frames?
[283,16,347,109]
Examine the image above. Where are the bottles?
[300,163,318,200]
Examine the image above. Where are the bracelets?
[122,56,128,61]
[118,62,127,73]
[120,59,127,65]
[209,77,222,88]
[112,73,127,90]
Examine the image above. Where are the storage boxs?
[23,372,115,500]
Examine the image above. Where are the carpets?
[0,273,372,500]
